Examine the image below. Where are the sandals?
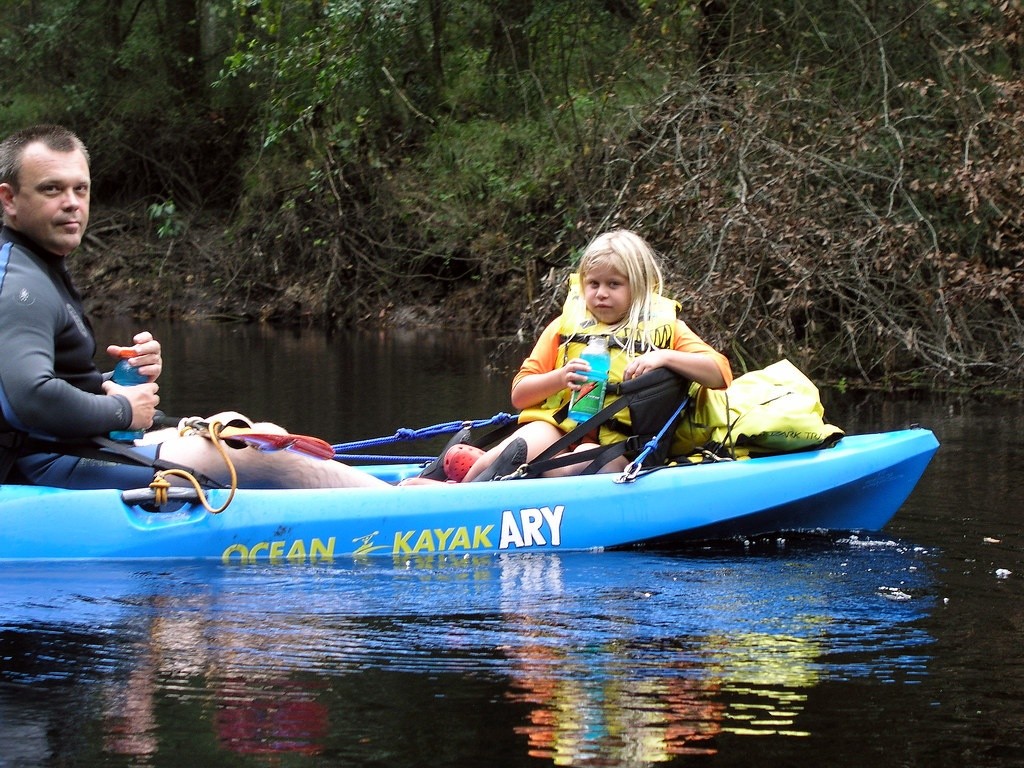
[396,477,446,486]
[443,444,486,483]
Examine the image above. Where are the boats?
[0,426,940,566]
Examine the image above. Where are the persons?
[394,229,732,487]
[0,126,392,490]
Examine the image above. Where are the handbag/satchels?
[669,358,846,459]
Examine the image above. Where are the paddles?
[149,408,337,462]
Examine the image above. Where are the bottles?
[108,348,149,439]
[567,334,610,423]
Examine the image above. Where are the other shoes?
[470,437,528,482]
[416,429,471,483]
